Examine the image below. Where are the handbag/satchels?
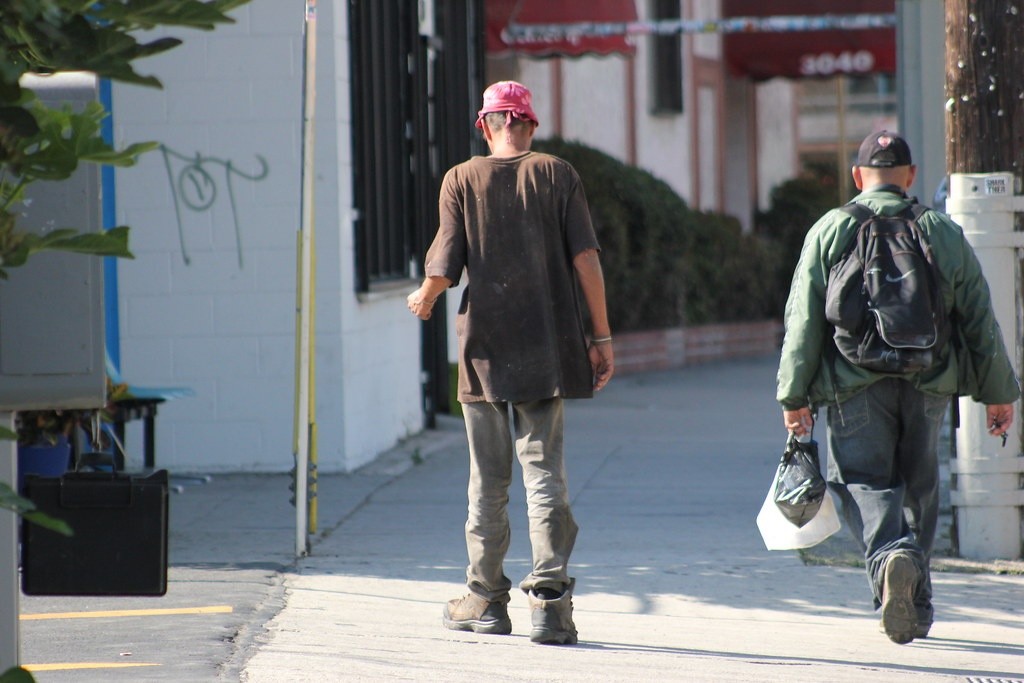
[755,415,842,551]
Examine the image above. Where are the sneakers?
[527,590,578,646]
[442,591,513,634]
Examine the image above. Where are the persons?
[407,81,614,646]
[776,130,1019,644]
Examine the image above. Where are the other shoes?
[878,549,934,645]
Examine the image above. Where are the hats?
[856,129,913,168]
[475,80,540,128]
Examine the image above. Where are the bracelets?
[590,337,612,342]
[418,289,437,305]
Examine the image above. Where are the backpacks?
[825,203,946,374]
